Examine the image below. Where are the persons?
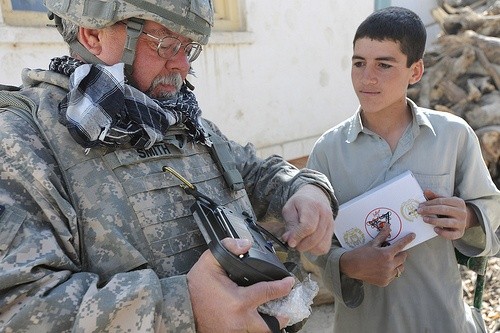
[0,0,338,333]
[303,6,500,333]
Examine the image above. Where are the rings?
[396,267,401,278]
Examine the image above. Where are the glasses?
[116,20,203,63]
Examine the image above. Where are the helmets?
[43,0,214,46]
